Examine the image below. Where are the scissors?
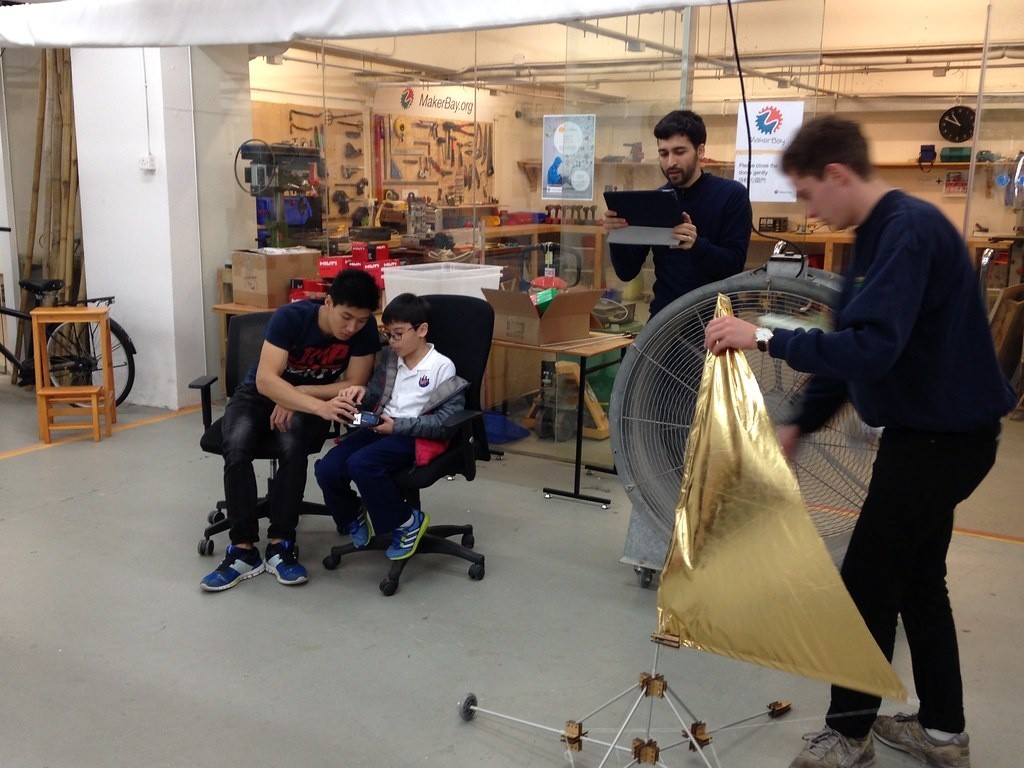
[337,120,363,131]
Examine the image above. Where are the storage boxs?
[481,288,607,347]
[380,262,504,306]
[941,147,972,162]
[991,290,1024,382]
[232,243,400,307]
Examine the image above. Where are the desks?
[377,205,494,230]
[213,303,382,395]
[324,246,523,291]
[481,225,1014,291]
[490,331,635,509]
[29,307,117,438]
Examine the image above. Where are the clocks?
[939,106,975,142]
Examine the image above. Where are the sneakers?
[263,541,308,585]
[347,497,372,550]
[385,508,429,561]
[199,545,267,590]
[872,711,970,768]
[788,723,876,768]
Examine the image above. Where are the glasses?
[380,323,421,341]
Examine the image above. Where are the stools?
[38,386,104,444]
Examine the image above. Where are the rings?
[689,236,690,240]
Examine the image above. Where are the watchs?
[755,326,774,352]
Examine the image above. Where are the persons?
[602,110,752,481]
[547,156,566,186]
[704,113,1015,768]
[317,293,470,562]
[200,269,383,593]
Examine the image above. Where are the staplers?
[973,223,988,232]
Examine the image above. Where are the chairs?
[188,293,495,597]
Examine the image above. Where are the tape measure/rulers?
[382,180,440,185]
[390,159,403,178]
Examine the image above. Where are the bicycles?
[0,224,137,412]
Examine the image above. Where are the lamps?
[353,41,1024,84]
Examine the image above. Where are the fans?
[608,255,885,589]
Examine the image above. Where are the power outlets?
[139,155,155,171]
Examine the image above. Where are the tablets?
[603,189,685,228]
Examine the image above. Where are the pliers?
[443,121,474,136]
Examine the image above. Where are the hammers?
[450,136,464,167]
